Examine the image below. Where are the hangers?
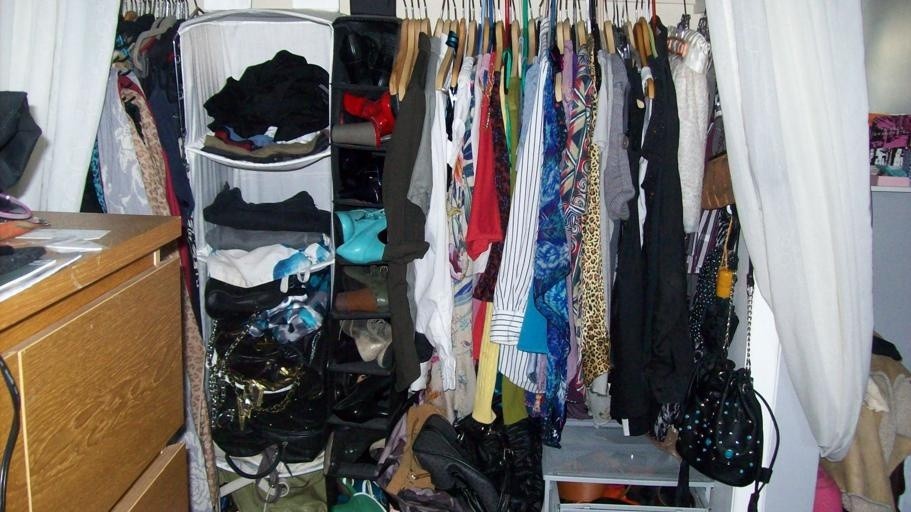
[111,0,206,73]
[387,1,659,112]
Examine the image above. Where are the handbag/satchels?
[212,335,331,512]
[458,405,545,512]
[675,357,764,487]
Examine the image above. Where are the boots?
[385,405,445,495]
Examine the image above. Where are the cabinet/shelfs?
[175,6,402,511]
[1,207,191,511]
[542,420,716,511]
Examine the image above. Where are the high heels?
[331,90,397,148]
[334,207,387,266]
[330,467,388,512]
[334,266,389,314]
[332,319,393,369]
[339,152,382,203]
[333,373,391,424]
[334,429,385,464]
[342,32,394,86]
